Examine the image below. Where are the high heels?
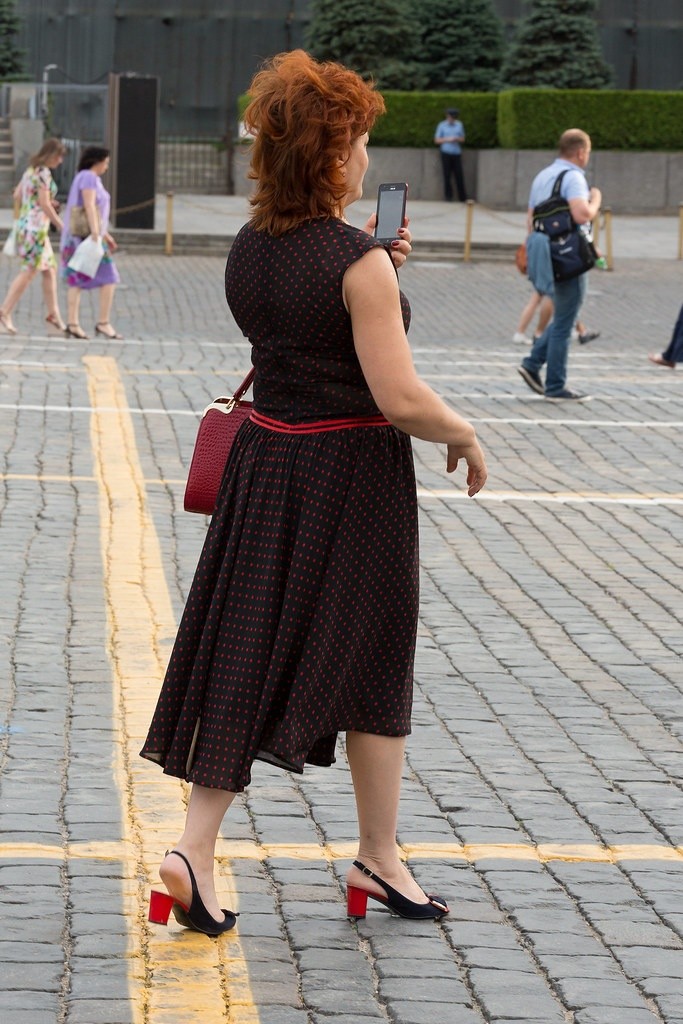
[64,323,90,339]
[148,850,238,933]
[345,859,450,919]
[94,321,124,339]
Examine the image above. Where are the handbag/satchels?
[68,234,105,279]
[184,364,256,513]
[2,222,18,258]
[71,202,101,236]
[515,240,528,274]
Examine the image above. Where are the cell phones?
[374,183,408,245]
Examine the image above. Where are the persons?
[434,110,467,201]
[0,138,67,334]
[516,129,601,401]
[512,290,601,344]
[131,48,488,933]
[648,303,683,368]
[60,147,124,339]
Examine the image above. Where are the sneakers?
[519,366,544,394]
[544,388,592,403]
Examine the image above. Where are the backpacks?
[531,173,600,283]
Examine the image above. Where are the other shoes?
[580,330,602,344]
[0,310,18,335]
[46,315,68,332]
[648,353,676,369]
[512,332,533,345]
